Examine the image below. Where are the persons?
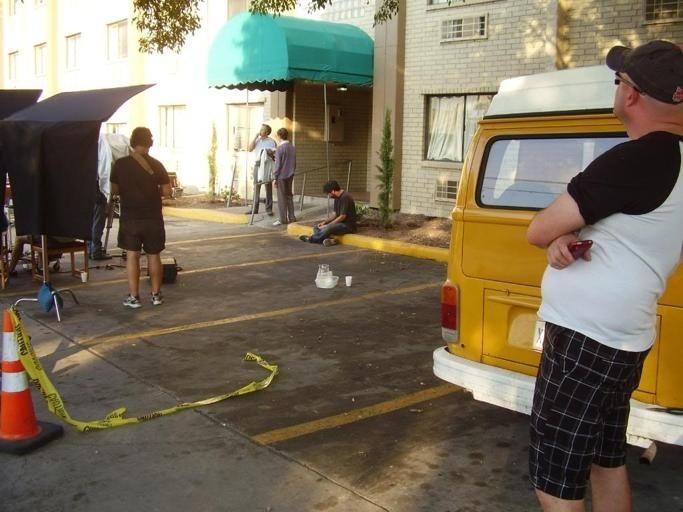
[300,180,356,246]
[245,124,277,216]
[110,126,171,309]
[87,135,117,259]
[6,235,75,277]
[272,127,296,227]
[525,41,683,512]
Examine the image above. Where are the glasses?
[614,72,646,97]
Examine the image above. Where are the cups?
[345,276,352,287]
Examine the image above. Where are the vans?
[427,63,681,468]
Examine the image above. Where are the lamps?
[336,85,348,92]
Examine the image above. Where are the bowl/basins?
[315,276,339,289]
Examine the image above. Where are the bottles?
[316,264,333,281]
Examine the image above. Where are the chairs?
[31,240,88,287]
[0,232,12,290]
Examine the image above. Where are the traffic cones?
[0,306,64,460]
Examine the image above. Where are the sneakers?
[322,238,337,247]
[150,289,163,306]
[123,295,141,308]
[91,253,111,260]
[299,234,311,243]
[245,210,296,226]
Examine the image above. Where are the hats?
[604,40,683,110]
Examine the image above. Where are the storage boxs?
[160,258,182,284]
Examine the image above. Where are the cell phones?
[568,239,593,260]
